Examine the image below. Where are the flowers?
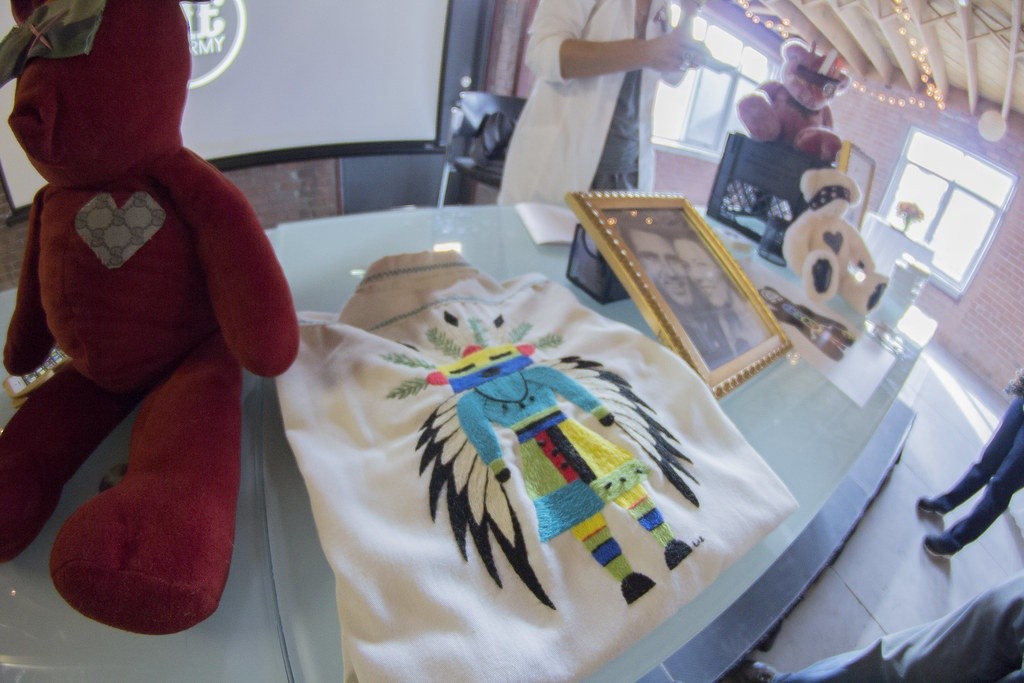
[896,202,925,232]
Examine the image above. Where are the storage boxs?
[706,133,833,259]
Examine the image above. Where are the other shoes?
[924,537,953,559]
[918,497,945,517]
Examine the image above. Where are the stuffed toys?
[782,170,889,314]
[738,41,849,162]
[0,0,299,634]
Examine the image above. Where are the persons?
[723,372,1024,683]
[621,214,773,371]
[497,0,705,204]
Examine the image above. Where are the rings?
[683,50,693,60]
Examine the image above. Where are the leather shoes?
[724,659,777,683]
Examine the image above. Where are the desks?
[0,201,919,683]
[861,211,935,281]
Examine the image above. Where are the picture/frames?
[836,140,878,235]
[566,191,794,401]
